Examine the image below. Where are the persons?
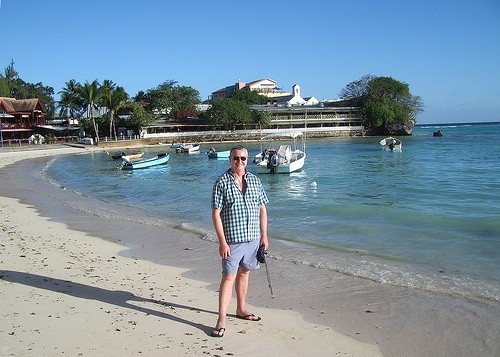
[211,145,269,337]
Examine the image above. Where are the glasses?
[234,157,246,161]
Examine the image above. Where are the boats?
[112,152,144,161]
[266,130,306,174]
[175,143,200,153]
[116,152,170,170]
[433,130,444,137]
[254,129,291,166]
[379,137,403,153]
[207,147,230,158]
[170,143,185,148]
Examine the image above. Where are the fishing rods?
[260,246,275,299]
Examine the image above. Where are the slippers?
[237,313,261,321]
[211,328,225,337]
